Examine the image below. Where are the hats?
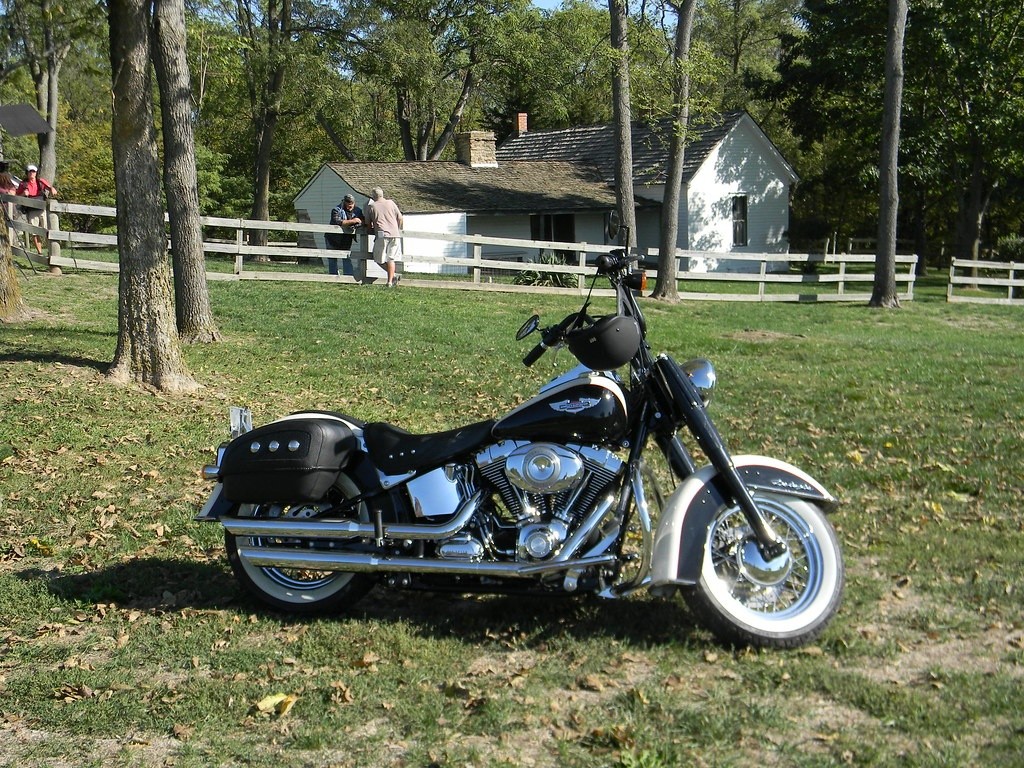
[27,166,37,172]
[342,194,355,204]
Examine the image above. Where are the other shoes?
[392,272,402,286]
[385,282,392,288]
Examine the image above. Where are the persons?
[16,165,58,257]
[323,193,364,275]
[0,160,16,247]
[364,186,404,288]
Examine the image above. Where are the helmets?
[567,315,641,371]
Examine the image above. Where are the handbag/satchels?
[23,195,46,211]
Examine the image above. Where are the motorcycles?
[194,212,845,650]
